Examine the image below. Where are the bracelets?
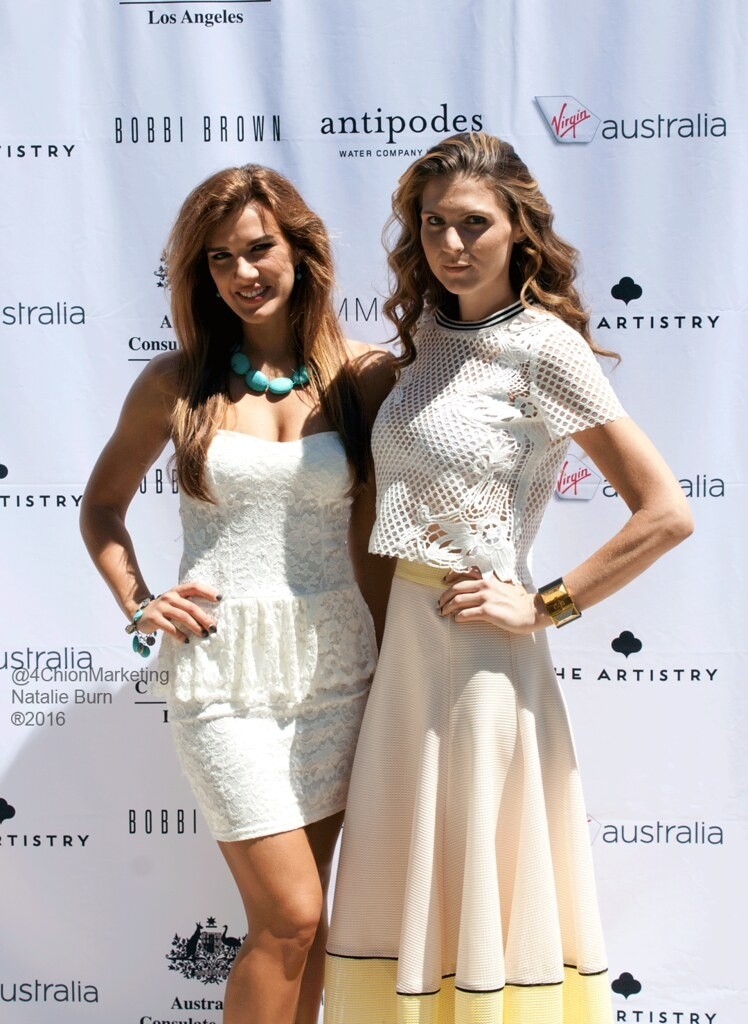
[126,593,161,657]
[536,577,583,630]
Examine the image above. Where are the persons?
[331,128,701,1024]
[77,163,398,1024]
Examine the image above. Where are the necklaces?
[228,332,313,394]
